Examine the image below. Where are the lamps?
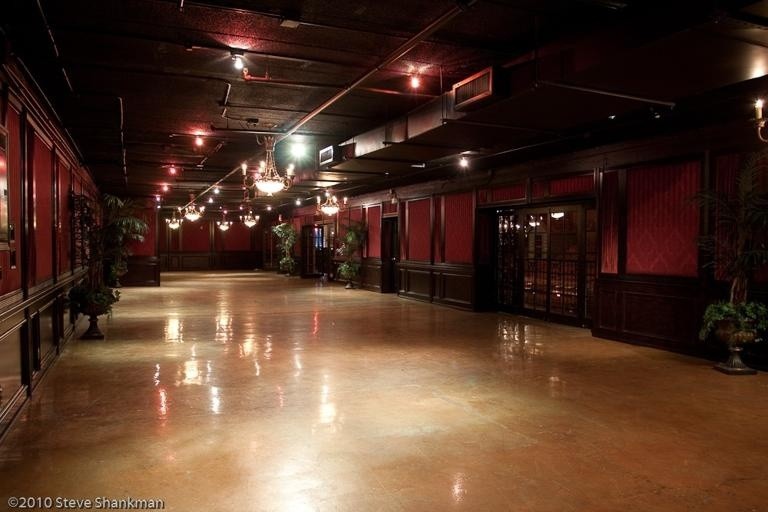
[315,190,348,216]
[240,122,296,197]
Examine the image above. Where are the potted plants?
[270,221,300,277]
[68,286,122,339]
[698,300,768,376]
[333,216,370,289]
[103,193,150,288]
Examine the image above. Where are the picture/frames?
[0,124,12,252]
